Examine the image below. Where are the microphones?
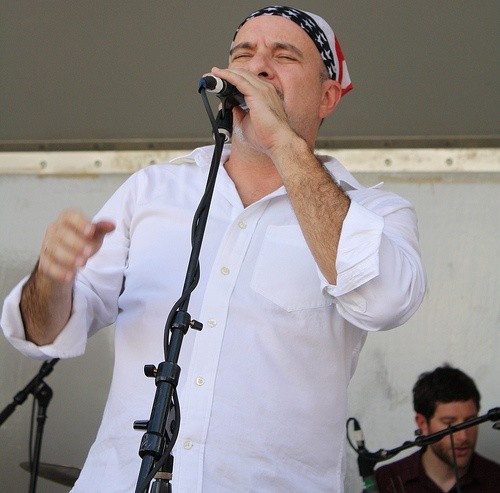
[352,420,379,493]
[201,75,250,112]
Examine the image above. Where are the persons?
[0,6,425,493]
[362,362,500,493]
